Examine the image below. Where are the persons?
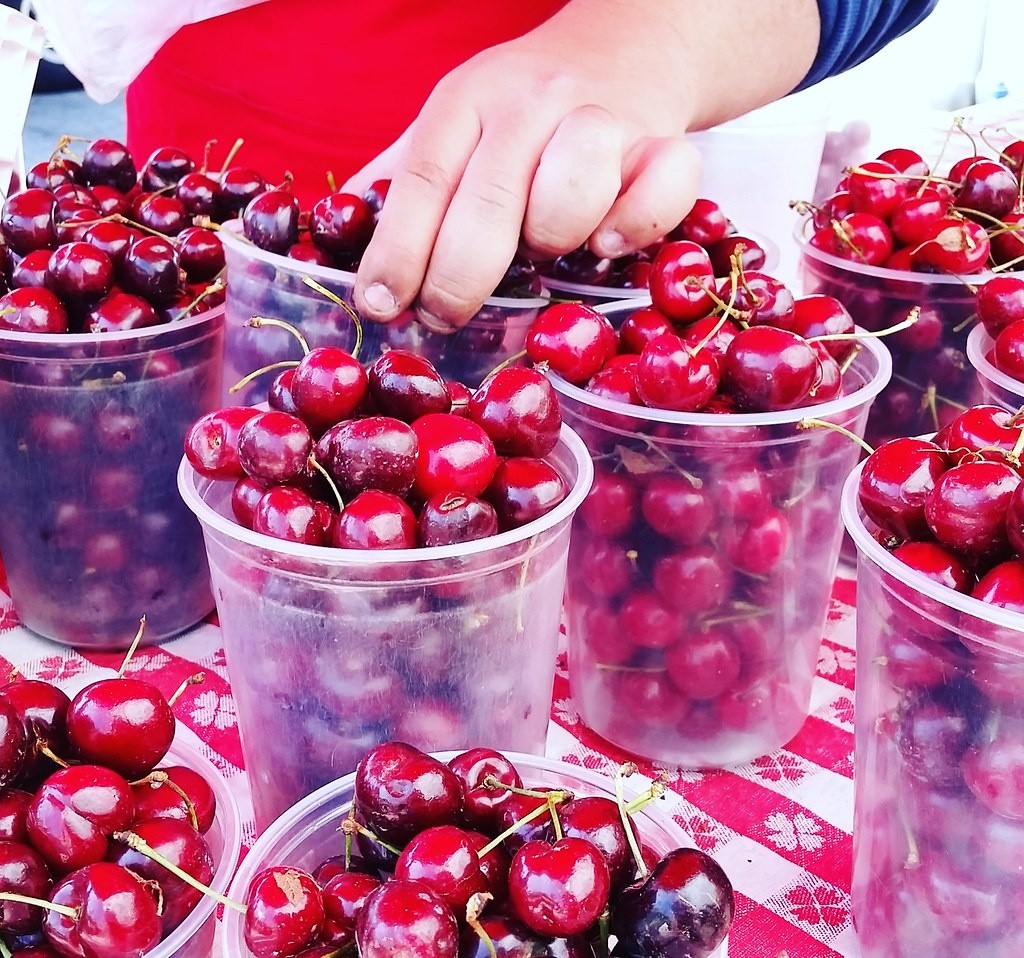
[0,1,941,343]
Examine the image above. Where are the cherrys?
[0,139,1023,958]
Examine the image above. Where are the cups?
[220,750,728,958]
[840,432,1024,958]
[0,734,240,958]
[791,209,1023,568]
[0,300,228,652]
[175,401,595,840]
[532,296,892,768]
[217,219,651,408]
